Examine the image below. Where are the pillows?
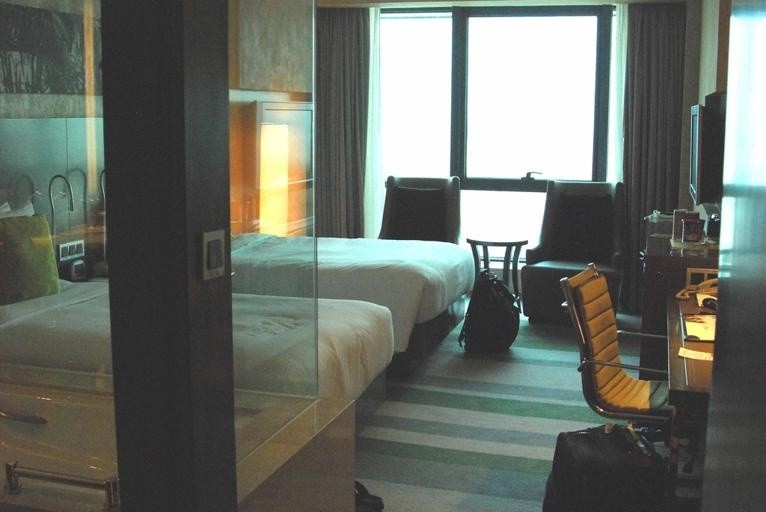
[0,212,57,304]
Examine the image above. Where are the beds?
[0,200,394,512]
[232,231,478,362]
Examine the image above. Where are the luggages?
[541,421,666,512]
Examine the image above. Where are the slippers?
[353,479,383,510]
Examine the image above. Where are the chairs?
[521,179,628,328]
[558,262,694,455]
[378,176,461,245]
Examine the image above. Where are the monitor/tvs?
[688,104,713,206]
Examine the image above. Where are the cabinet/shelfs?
[638,212,718,379]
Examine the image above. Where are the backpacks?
[457,268,520,354]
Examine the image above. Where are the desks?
[666,282,721,512]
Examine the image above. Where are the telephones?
[675,278,721,300]
